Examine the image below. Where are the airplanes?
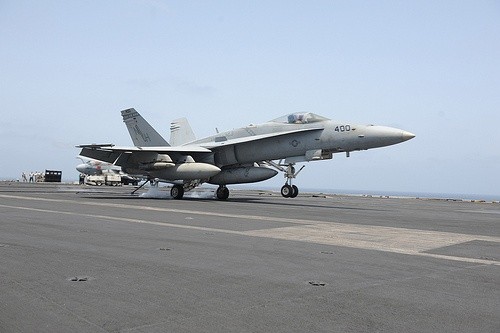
[75,155,147,178]
[75,106,417,201]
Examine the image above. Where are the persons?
[22,169,45,182]
